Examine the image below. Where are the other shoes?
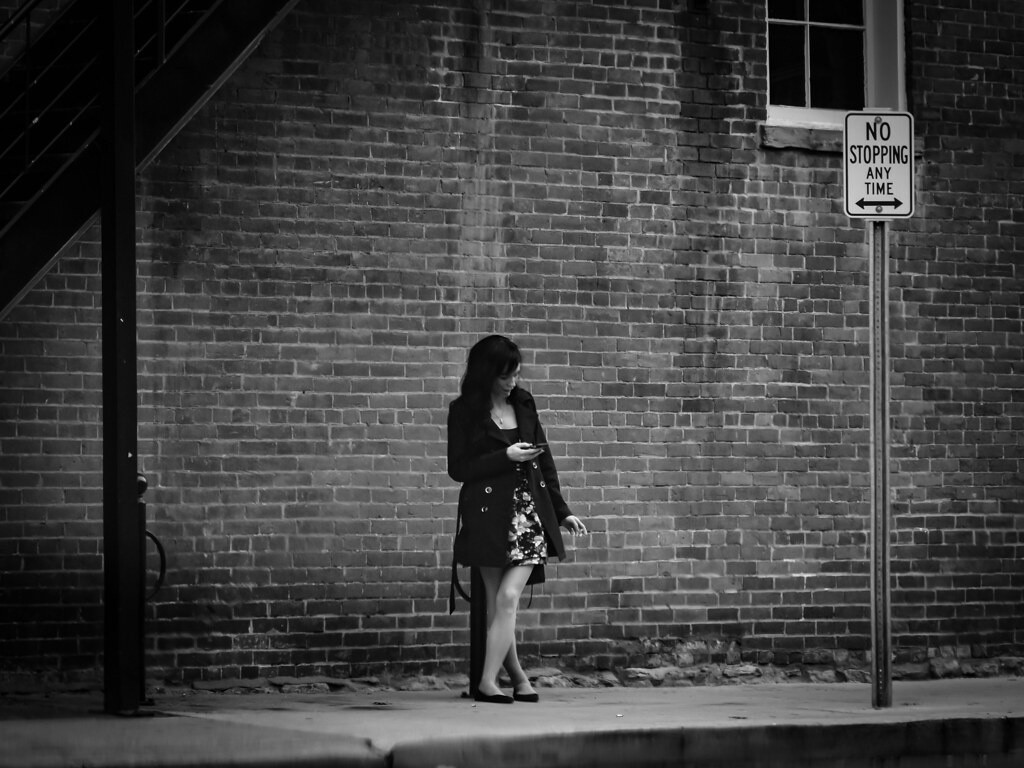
[474,688,514,703]
[513,688,539,702]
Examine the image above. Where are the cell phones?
[532,443,548,449]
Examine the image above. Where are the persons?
[447,334,588,700]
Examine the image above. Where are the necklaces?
[493,406,505,425]
